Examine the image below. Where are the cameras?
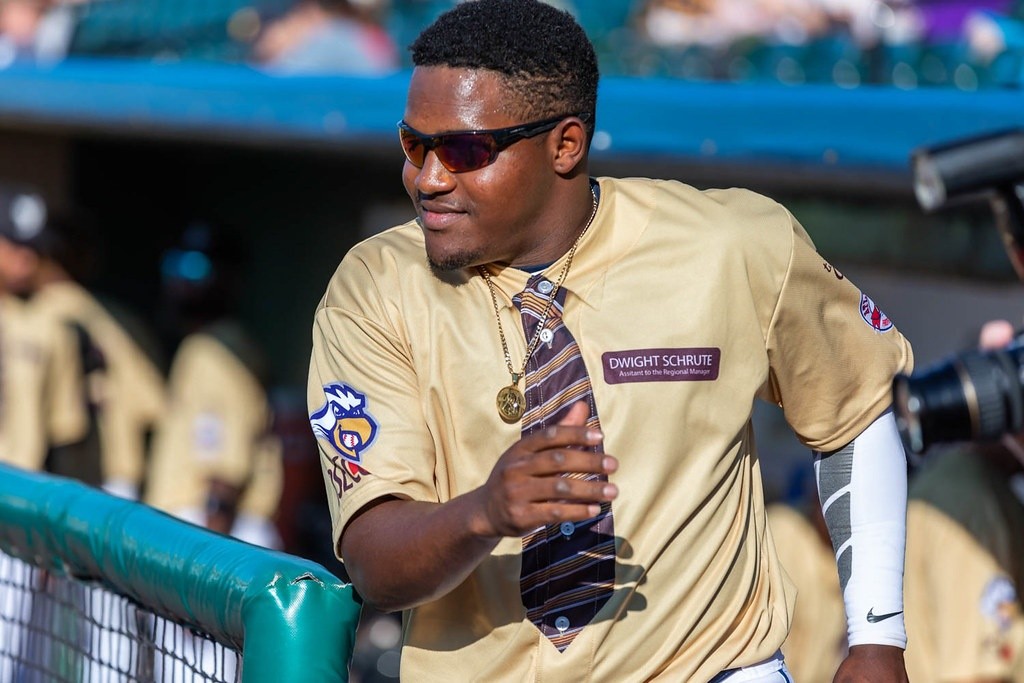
[892,330,1024,455]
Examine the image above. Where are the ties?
[513,277,616,656]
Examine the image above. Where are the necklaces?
[480,182,598,423]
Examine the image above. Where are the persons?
[0,0,1024,683]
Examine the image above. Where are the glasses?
[399,111,579,173]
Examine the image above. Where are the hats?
[0,184,54,254]
